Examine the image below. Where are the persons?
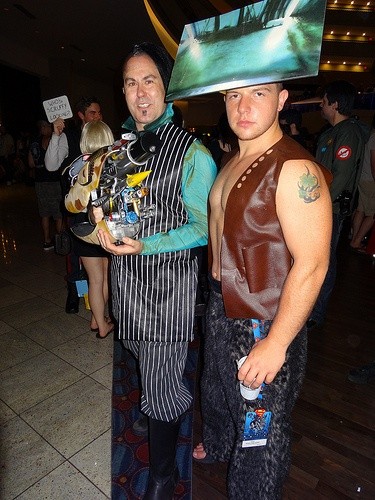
[190,79,332,499]
[307,86,364,328]
[44,98,104,314]
[96,43,217,500]
[349,121,375,250]
[60,120,116,337]
[346,205,375,385]
[0,122,27,186]
[170,104,318,166]
[28,119,69,250]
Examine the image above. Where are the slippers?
[96,322,115,340]
[91,316,108,332]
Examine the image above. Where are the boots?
[143,418,180,500]
[64,274,80,314]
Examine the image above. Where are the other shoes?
[43,240,55,250]
[344,363,375,385]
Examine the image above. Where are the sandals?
[193,442,217,466]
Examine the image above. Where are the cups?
[237,356,262,400]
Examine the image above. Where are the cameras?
[334,190,353,216]
